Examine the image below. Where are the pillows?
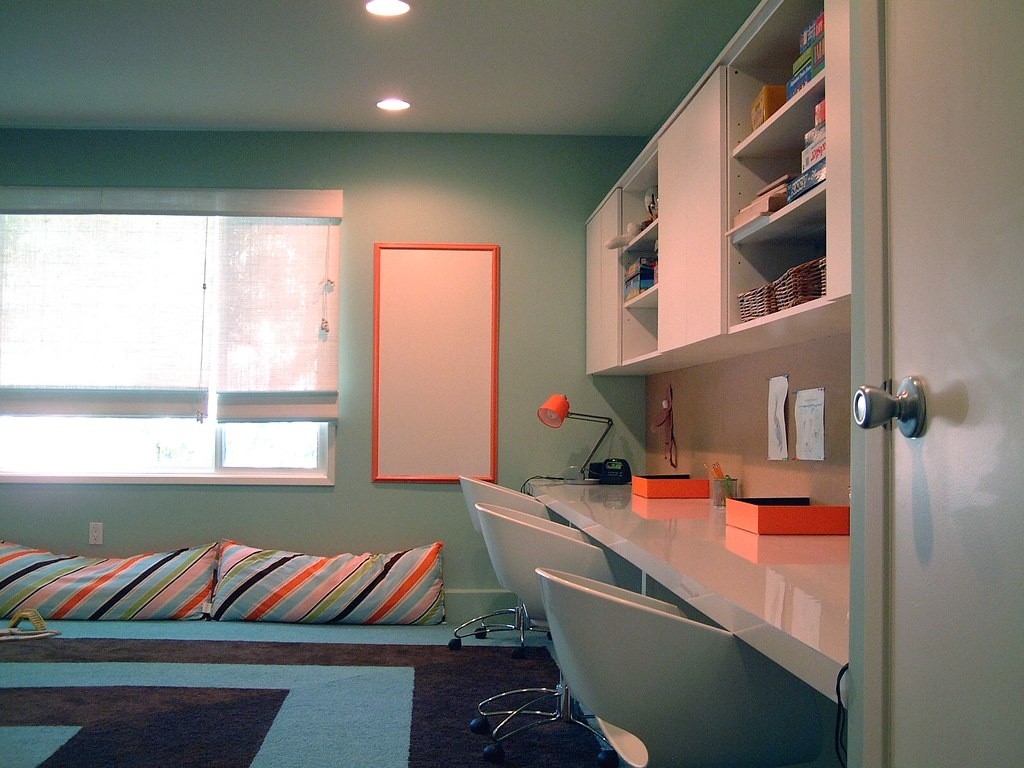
[215,539,445,626]
[0,540,218,623]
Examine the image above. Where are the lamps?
[536,395,615,483]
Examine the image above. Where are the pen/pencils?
[703,462,733,498]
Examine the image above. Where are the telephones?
[588,458,631,485]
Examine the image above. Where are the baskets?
[738,281,779,323]
[773,256,826,311]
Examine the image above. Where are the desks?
[523,477,851,710]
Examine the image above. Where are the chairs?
[446,475,553,652]
[533,564,826,768]
[468,501,620,767]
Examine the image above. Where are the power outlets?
[89,521,104,547]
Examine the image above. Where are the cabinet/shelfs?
[659,65,780,365]
[621,147,692,383]
[728,2,851,352]
[584,190,666,383]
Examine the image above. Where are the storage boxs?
[632,472,710,499]
[750,9,825,203]
[725,495,850,534]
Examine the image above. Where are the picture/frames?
[372,243,501,485]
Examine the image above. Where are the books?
[725,172,800,237]
